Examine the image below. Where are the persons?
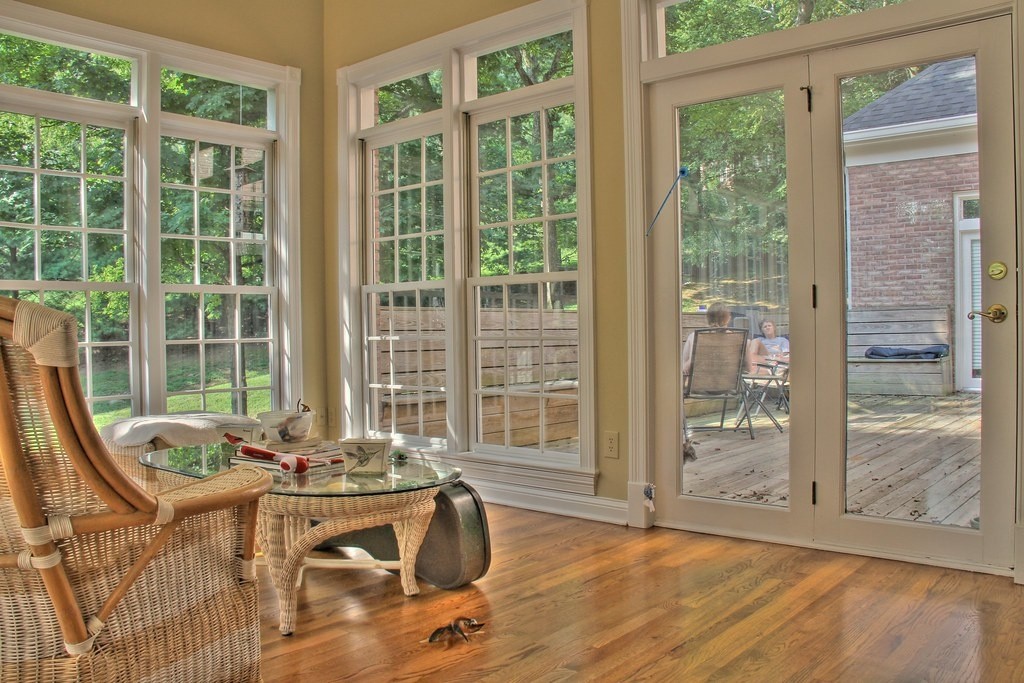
[681,300,736,396]
[742,314,789,376]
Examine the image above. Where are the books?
[226,438,345,472]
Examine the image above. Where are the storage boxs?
[341,438,393,474]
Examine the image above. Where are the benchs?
[735,310,954,397]
[378,305,739,448]
[99,410,264,499]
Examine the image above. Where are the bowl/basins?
[256,410,316,443]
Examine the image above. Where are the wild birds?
[222,433,249,447]
[344,446,382,473]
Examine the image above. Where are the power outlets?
[603,430,619,460]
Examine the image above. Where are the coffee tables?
[138,437,463,639]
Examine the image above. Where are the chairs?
[681,327,755,443]
[752,333,789,341]
[734,361,790,433]
[735,376,790,425]
[0,296,275,683]
